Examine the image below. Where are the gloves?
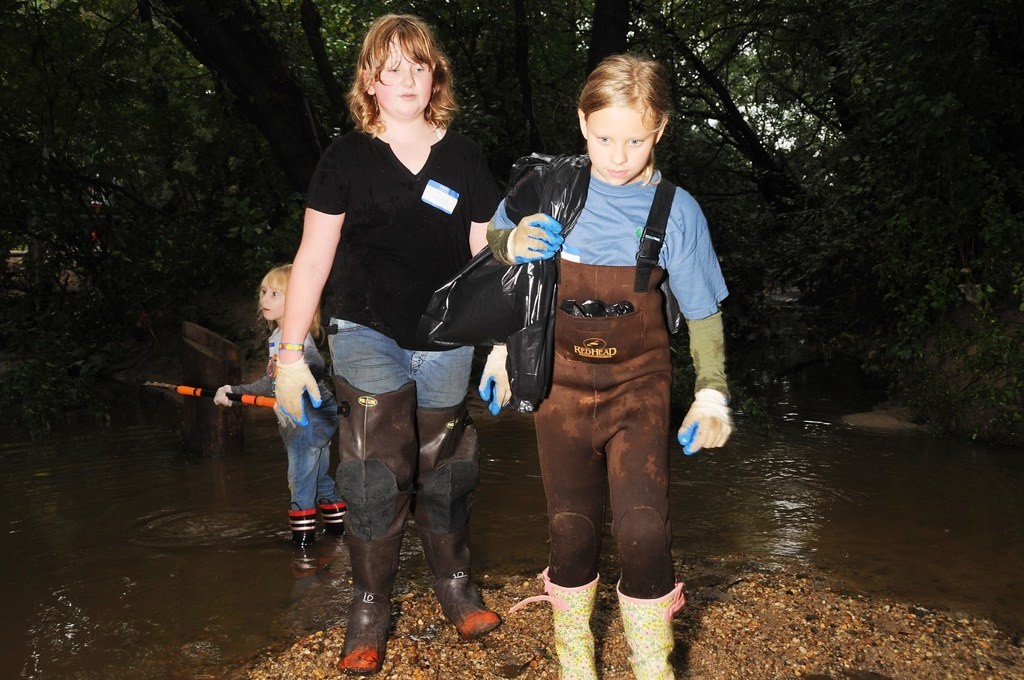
[213,384,233,407]
[275,355,322,428]
[478,345,512,415]
[508,213,563,266]
[678,389,733,456]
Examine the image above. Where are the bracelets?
[278,343,304,352]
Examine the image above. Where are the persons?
[273,14,513,675]
[214,264,346,550]
[487,55,735,680]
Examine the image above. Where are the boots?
[330,365,417,674]
[411,400,501,641]
[616,578,686,679]
[319,503,347,537]
[508,566,599,680]
[288,507,317,550]
[292,550,319,577]
